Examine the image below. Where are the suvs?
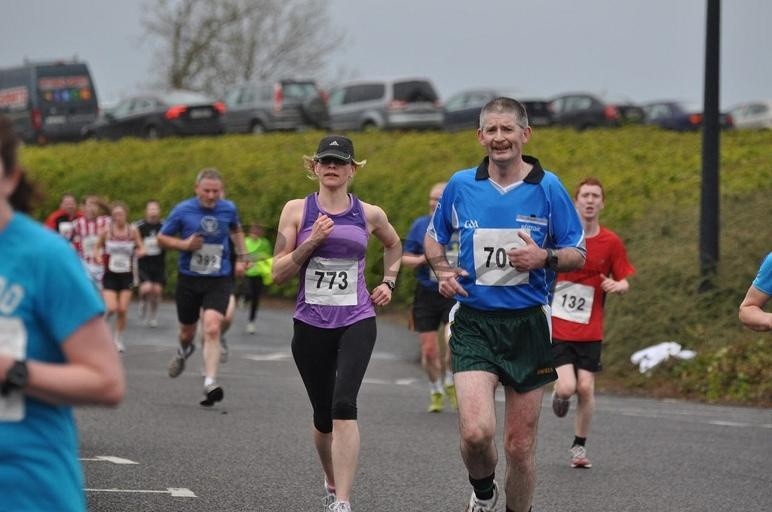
[0,54,101,149]
[315,76,447,135]
[207,78,332,134]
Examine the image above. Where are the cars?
[79,88,226,140]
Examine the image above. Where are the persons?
[225,220,272,333]
[0,114,125,512]
[551,177,636,469]
[739,251,772,332]
[402,181,459,414]
[47,191,165,349]
[424,97,587,512]
[272,136,402,512]
[156,167,244,408]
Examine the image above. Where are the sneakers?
[429,392,445,413]
[329,499,351,511]
[444,381,458,413]
[552,392,569,417]
[322,476,336,512]
[569,445,592,469]
[465,480,499,511]
[199,385,224,407]
[113,302,159,352]
[247,323,256,334]
[169,345,196,377]
[220,339,228,362]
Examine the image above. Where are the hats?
[315,135,355,162]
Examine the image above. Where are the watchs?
[382,280,395,290]
[546,248,558,270]
[1,359,28,394]
